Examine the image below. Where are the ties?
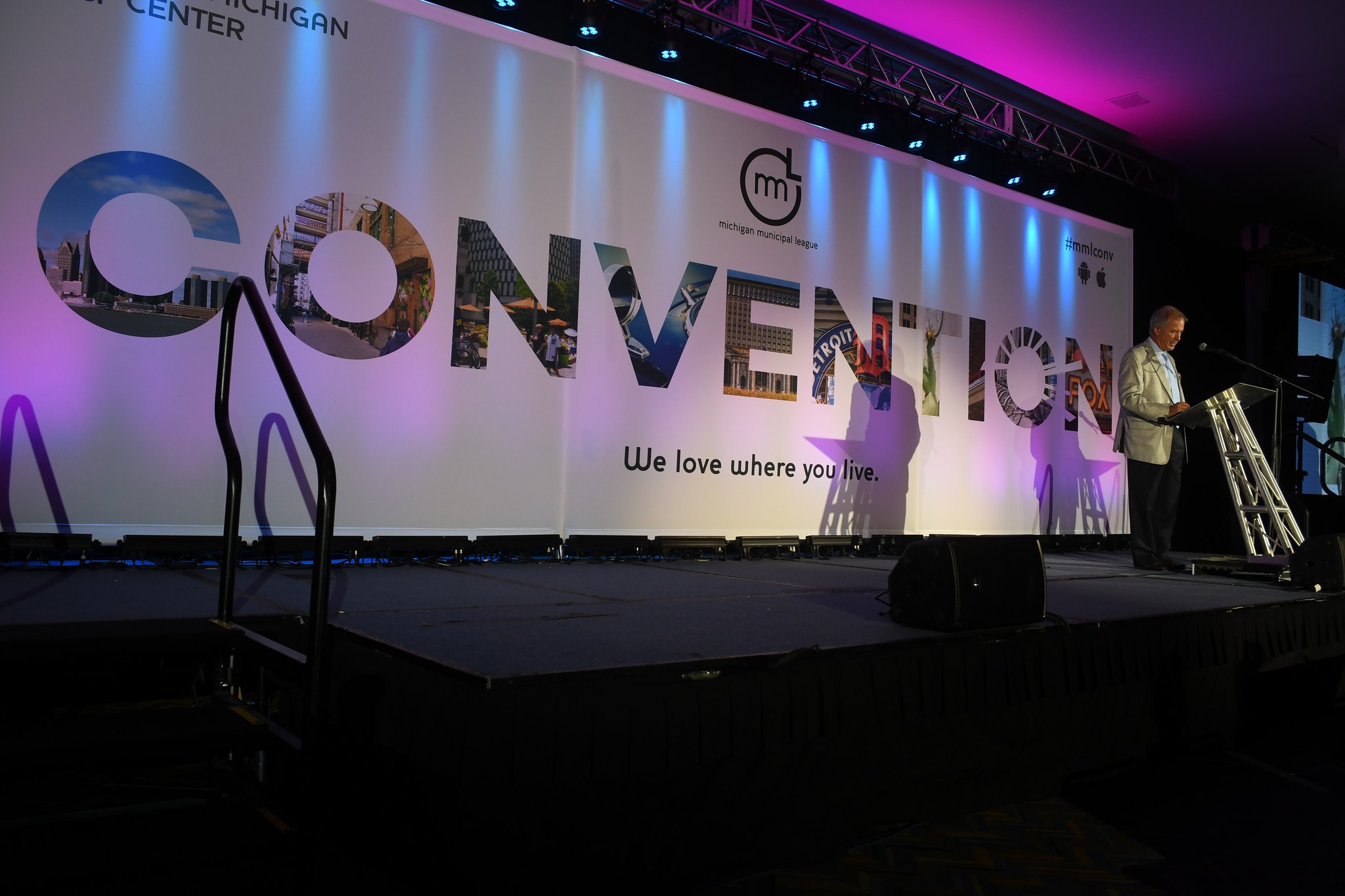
[1162,352,1181,429]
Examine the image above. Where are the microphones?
[1198,343,1232,355]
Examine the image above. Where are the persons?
[1112,305,1190,571]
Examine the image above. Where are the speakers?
[1289,533,1345,592]
[888,534,1047,632]
[1292,354,1336,424]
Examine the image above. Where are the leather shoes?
[1161,556,1187,570]
[1134,562,1167,570]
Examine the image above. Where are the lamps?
[941,125,969,164]
[1036,161,1058,198]
[492,0,519,11]
[896,106,926,151]
[574,0,602,39]
[800,83,821,111]
[656,26,682,62]
[853,92,879,132]
[995,152,1023,187]
